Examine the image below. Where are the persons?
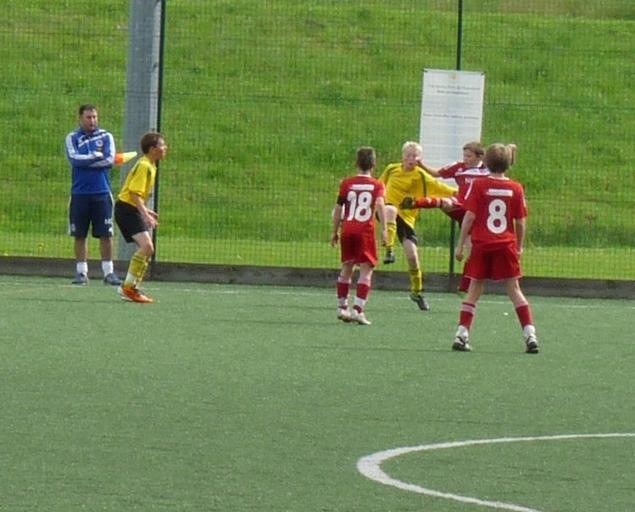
[115,133,167,302]
[397,141,488,227]
[64,104,122,286]
[451,142,541,354]
[374,140,458,312]
[329,146,387,326]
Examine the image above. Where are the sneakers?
[383,250,395,263]
[336,306,352,323]
[350,309,372,325]
[72,272,88,284]
[104,273,123,285]
[399,197,413,209]
[117,283,153,303]
[409,292,429,310]
[525,335,539,353]
[452,336,472,351]
